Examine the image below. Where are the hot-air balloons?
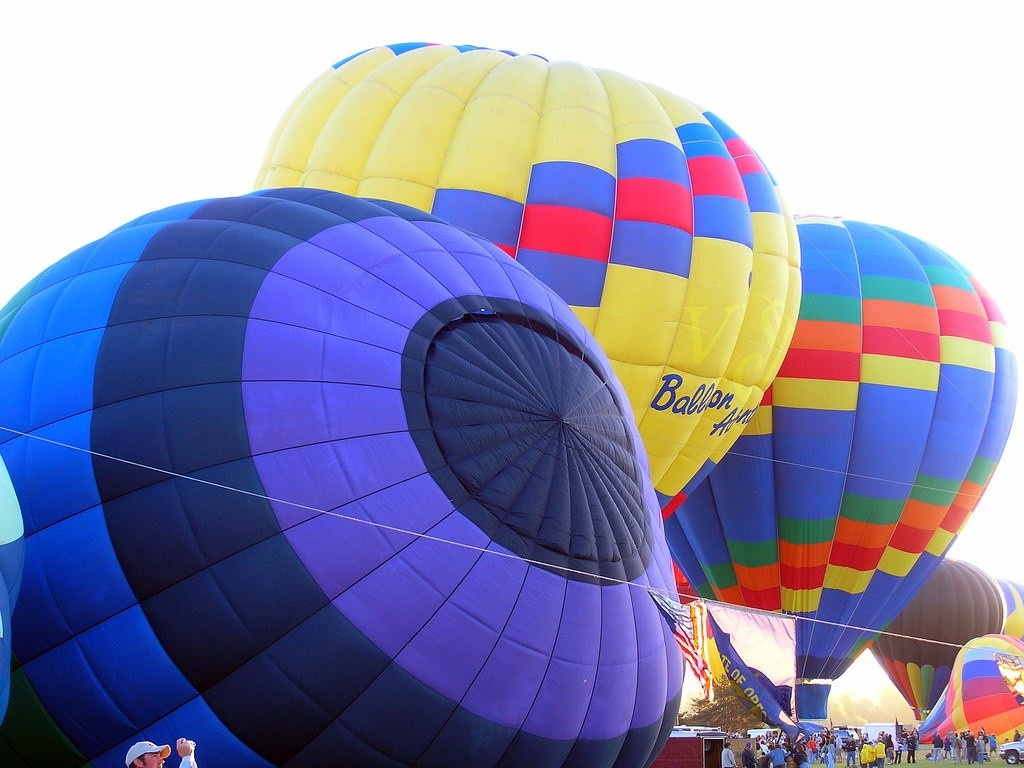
[993,577,1024,638]
[910,633,1024,749]
[864,555,1007,726]
[0,188,685,768]
[243,38,799,525]
[650,212,1017,764]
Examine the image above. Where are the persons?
[721,727,1024,768]
[125,737,198,768]
[995,655,1022,670]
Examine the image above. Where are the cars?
[846,730,860,746]
[829,730,850,751]
[999,738,1024,765]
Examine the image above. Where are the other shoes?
[888,762,891,764]
[851,765,854,768]
[846,765,849,768]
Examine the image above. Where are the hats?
[125,741,171,768]
[746,742,751,747]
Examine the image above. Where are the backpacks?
[746,753,755,764]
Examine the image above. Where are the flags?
[646,587,715,702]
